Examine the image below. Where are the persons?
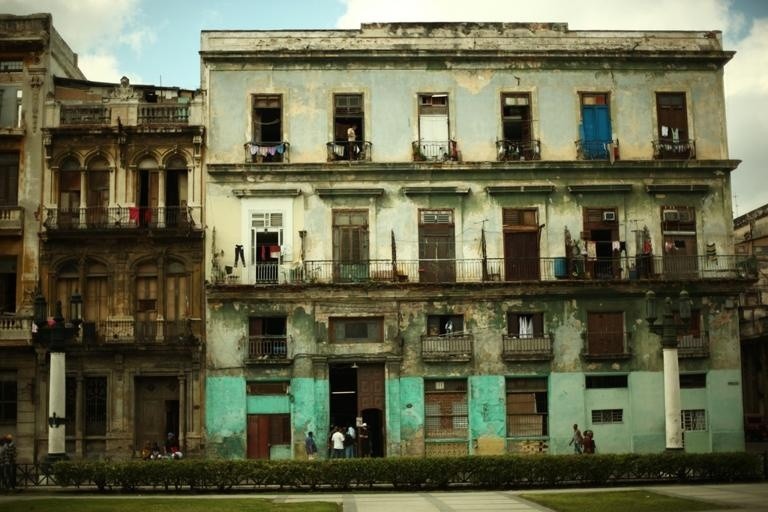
[304,430,318,460]
[582,429,596,453]
[152,440,160,452]
[568,424,582,455]
[327,421,373,459]
[170,445,182,463]
[344,128,360,158]
[141,438,153,461]
[444,318,455,335]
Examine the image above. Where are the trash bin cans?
[554,257,568,278]
